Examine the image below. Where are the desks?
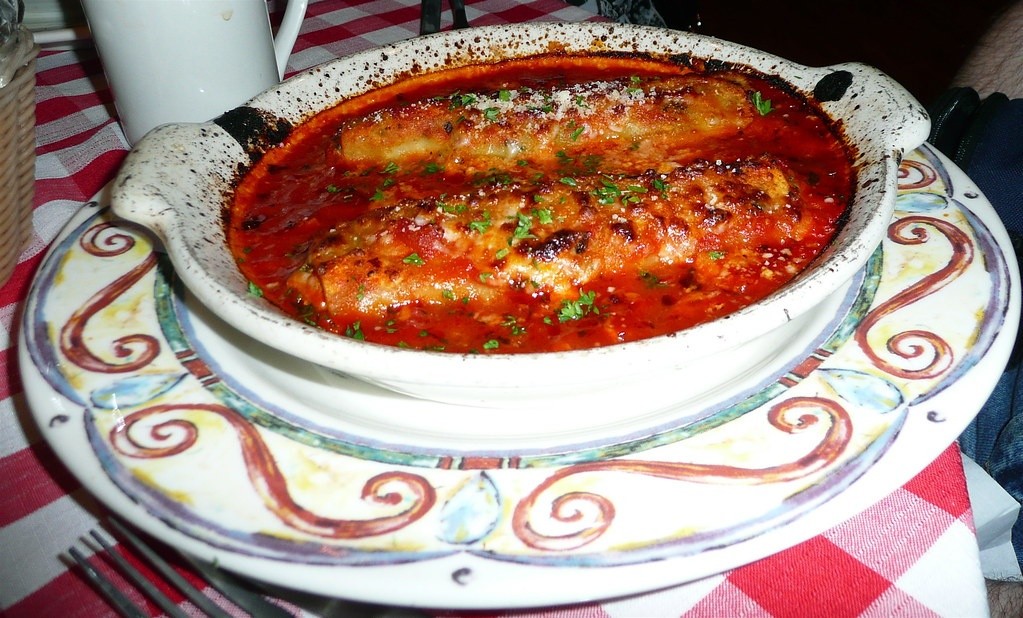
[0,1,988,618]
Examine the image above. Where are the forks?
[69,515,297,617]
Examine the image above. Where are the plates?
[17,142,1021,611]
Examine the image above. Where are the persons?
[926,0,1023,574]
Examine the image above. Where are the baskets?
[0,25,40,287]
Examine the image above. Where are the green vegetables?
[237,77,774,351]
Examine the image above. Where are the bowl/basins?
[110,21,932,411]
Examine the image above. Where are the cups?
[79,0,309,149]
